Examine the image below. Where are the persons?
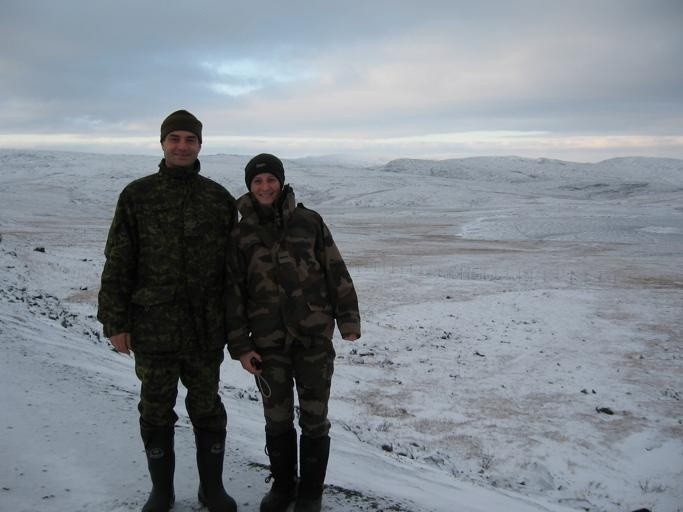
[224,152,361,511]
[98,110,239,511]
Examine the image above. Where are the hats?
[245,154,285,192]
[161,110,202,145]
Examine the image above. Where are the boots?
[193,427,237,512]
[260,428,298,512]
[141,428,175,512]
[293,435,330,512]
